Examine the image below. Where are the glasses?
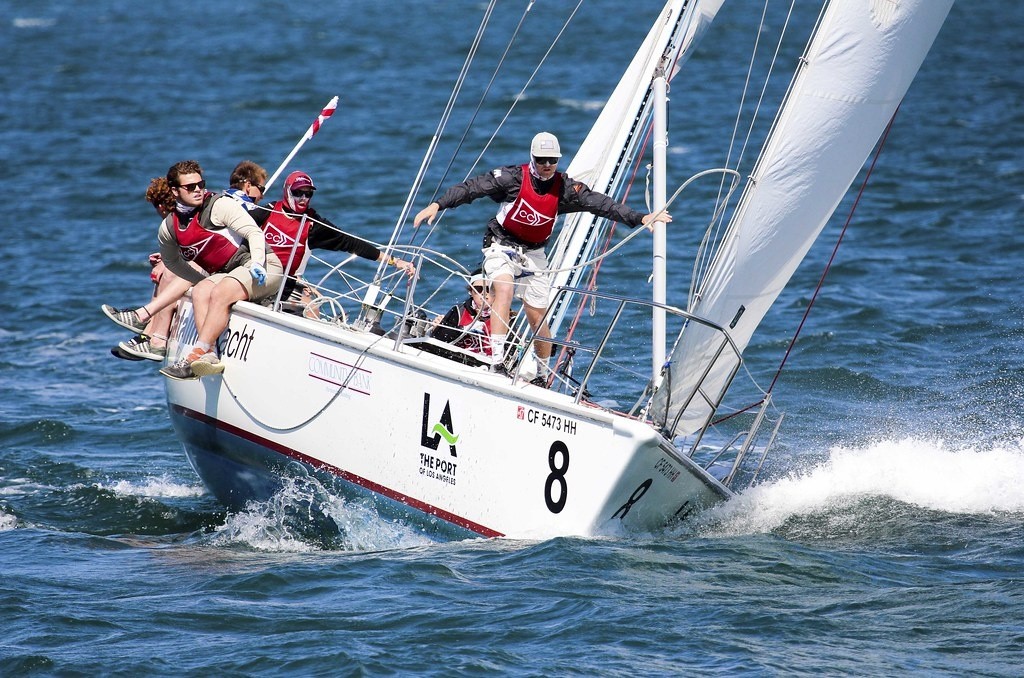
[533,155,558,165]
[239,179,265,195]
[175,180,205,191]
[291,189,313,198]
[473,286,489,293]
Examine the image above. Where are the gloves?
[250,262,268,288]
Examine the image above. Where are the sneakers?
[159,357,200,380]
[101,304,151,334]
[190,352,225,376]
[118,337,167,362]
[111,334,151,361]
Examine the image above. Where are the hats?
[532,132,562,157]
[290,176,316,190]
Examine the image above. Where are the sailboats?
[159,2,958,544]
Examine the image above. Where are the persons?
[412,132,672,390]
[247,172,420,284]
[102,161,284,381]
[431,266,521,359]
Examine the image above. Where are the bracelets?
[389,256,395,265]
[394,257,400,266]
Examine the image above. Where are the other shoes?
[528,373,548,388]
[490,363,507,377]
